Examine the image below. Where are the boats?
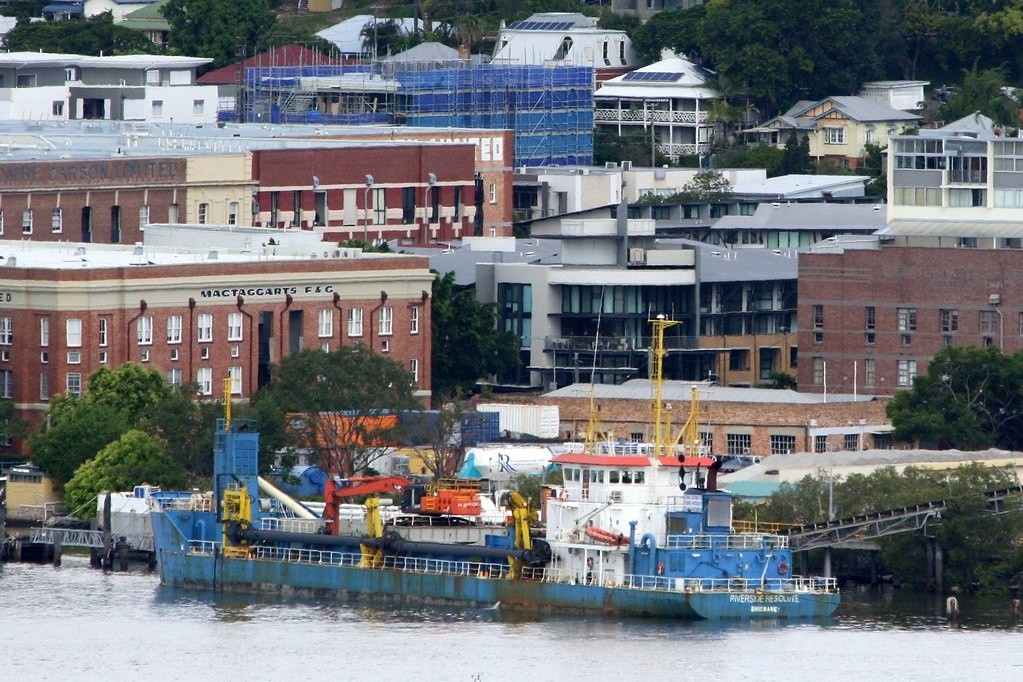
[145,306,843,623]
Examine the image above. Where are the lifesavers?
[777,562,789,574]
[587,557,594,568]
[657,562,665,574]
